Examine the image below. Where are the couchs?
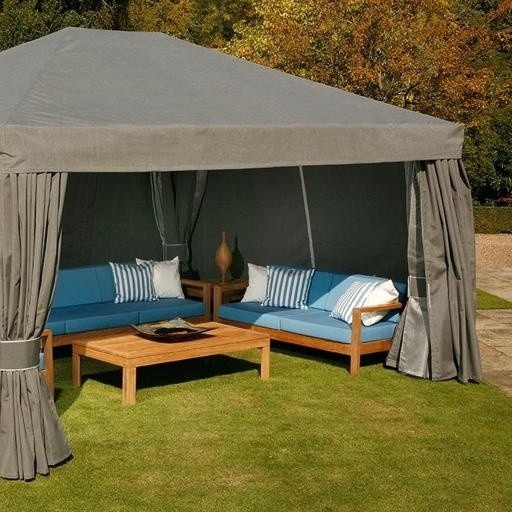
[35,329,54,407]
[39,255,213,348]
[213,263,406,376]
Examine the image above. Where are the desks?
[188,277,247,304]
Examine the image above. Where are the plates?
[130,316,219,341]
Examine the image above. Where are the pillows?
[107,259,159,304]
[329,281,377,325]
[260,264,315,310]
[135,255,185,299]
[241,262,267,302]
[359,279,400,326]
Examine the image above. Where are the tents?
[3,27,483,485]
